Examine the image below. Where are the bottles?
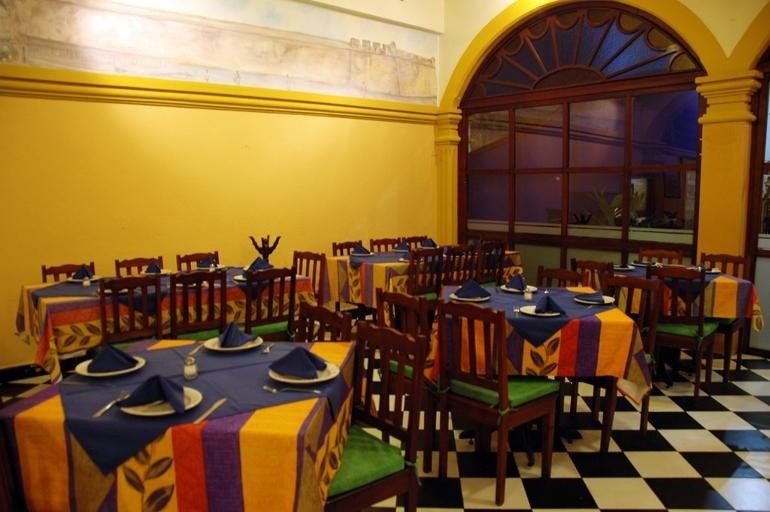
[522,285,533,301]
[208,263,216,272]
[182,356,198,380]
[82,275,91,289]
[267,361,340,383]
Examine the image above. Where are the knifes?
[185,335,206,357]
[192,396,230,426]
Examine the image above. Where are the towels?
[114,373,188,412]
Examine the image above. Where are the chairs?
[324,319,427,512]
[175,252,220,270]
[569,274,659,456]
[569,257,616,278]
[698,252,750,386]
[637,249,683,267]
[115,256,165,273]
[291,248,329,308]
[328,233,524,301]
[538,264,579,288]
[244,267,298,331]
[648,264,718,400]
[437,295,556,505]
[40,261,95,282]
[94,272,162,342]
[167,269,230,335]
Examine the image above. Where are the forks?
[260,382,321,397]
[90,387,126,421]
[263,339,275,356]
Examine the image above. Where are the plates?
[232,273,248,281]
[75,354,145,379]
[194,262,224,269]
[98,287,133,296]
[573,294,617,305]
[170,281,201,288]
[141,267,169,277]
[606,258,722,275]
[65,273,104,283]
[345,242,464,263]
[448,292,491,303]
[500,283,538,293]
[200,334,264,354]
[120,385,201,416]
[520,304,560,317]
